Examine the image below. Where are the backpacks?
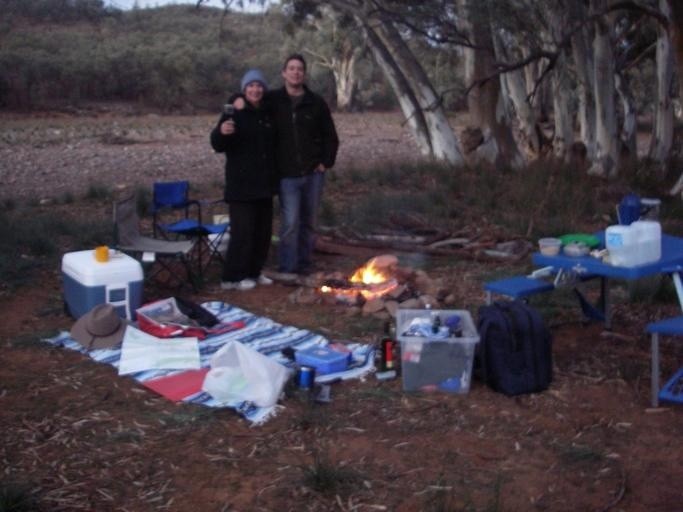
[474,300,552,394]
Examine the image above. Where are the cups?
[223,104,234,125]
[297,365,316,391]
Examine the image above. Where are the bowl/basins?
[538,238,561,255]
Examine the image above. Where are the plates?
[558,233,600,248]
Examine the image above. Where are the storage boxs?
[396,310,479,394]
[61,250,145,323]
[295,348,349,373]
[606,220,660,266]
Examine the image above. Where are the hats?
[240,71,267,93]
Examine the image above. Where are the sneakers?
[258,274,273,285]
[271,266,299,281]
[221,279,255,290]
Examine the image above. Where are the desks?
[531,234,683,336]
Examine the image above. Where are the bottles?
[424,303,431,316]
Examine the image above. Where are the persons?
[266,55,340,281]
[210,70,277,291]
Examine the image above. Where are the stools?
[645,315,683,406]
[483,277,554,306]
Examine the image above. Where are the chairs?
[154,181,230,288]
[113,193,198,294]
